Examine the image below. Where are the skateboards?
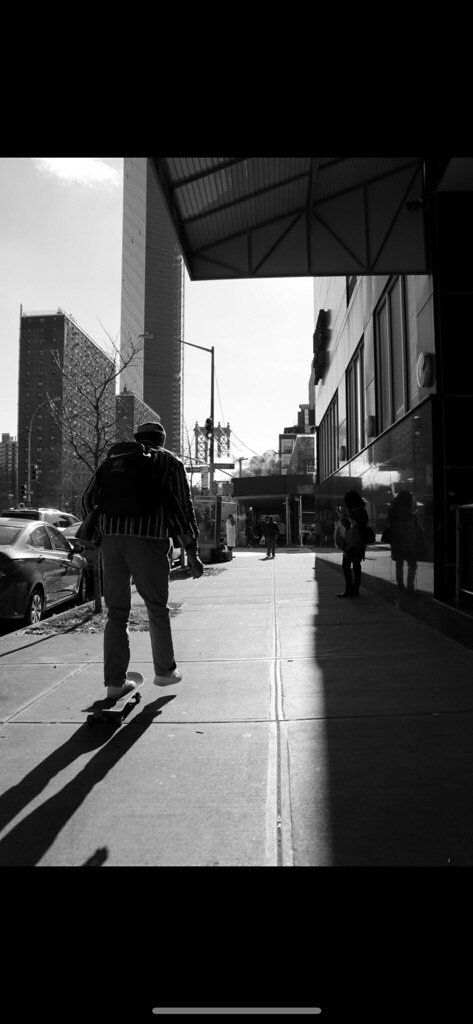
[80,671,144,724]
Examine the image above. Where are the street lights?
[25,395,63,507]
[138,332,215,493]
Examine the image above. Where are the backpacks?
[94,440,168,519]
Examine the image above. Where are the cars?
[58,521,188,598]
[0,516,88,626]
[2,507,76,532]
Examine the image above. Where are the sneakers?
[153,669,184,687]
[106,679,137,700]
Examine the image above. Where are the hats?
[132,422,166,439]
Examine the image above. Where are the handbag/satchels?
[351,526,376,546]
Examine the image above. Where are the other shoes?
[336,590,360,600]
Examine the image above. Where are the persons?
[82,423,204,699]
[245,515,285,547]
[336,491,369,598]
[387,489,422,595]
[264,516,280,558]
[226,514,236,552]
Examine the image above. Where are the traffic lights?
[206,417,213,438]
[31,463,39,481]
[20,484,28,499]
